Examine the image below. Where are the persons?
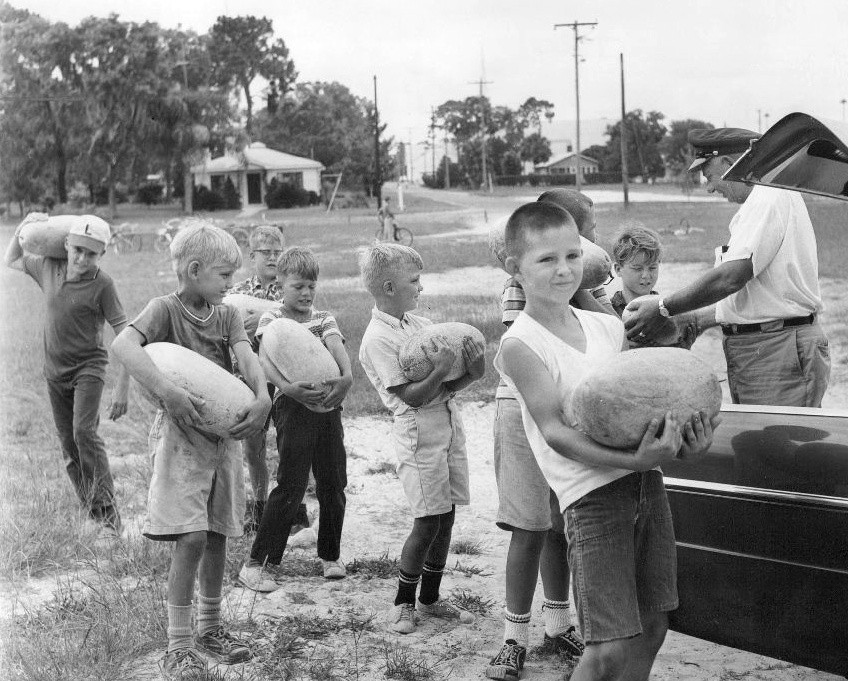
[623,128,832,408]
[611,229,700,351]
[359,243,486,633]
[502,203,723,681]
[238,247,355,592]
[110,226,270,681]
[487,188,622,679]
[5,211,130,529]
[381,196,396,242]
[225,227,302,533]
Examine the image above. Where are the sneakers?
[417,594,475,625]
[164,650,206,679]
[545,626,585,660]
[486,639,526,681]
[323,558,347,579]
[393,603,415,633]
[194,626,249,666]
[238,563,277,592]
[97,517,120,548]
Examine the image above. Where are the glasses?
[253,249,284,257]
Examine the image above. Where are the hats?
[687,128,763,174]
[67,215,111,254]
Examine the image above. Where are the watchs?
[659,295,675,318]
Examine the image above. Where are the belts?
[722,314,813,336]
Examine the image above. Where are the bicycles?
[154,217,250,251]
[111,224,142,253]
[375,215,414,247]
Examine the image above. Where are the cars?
[658,111,848,680]
